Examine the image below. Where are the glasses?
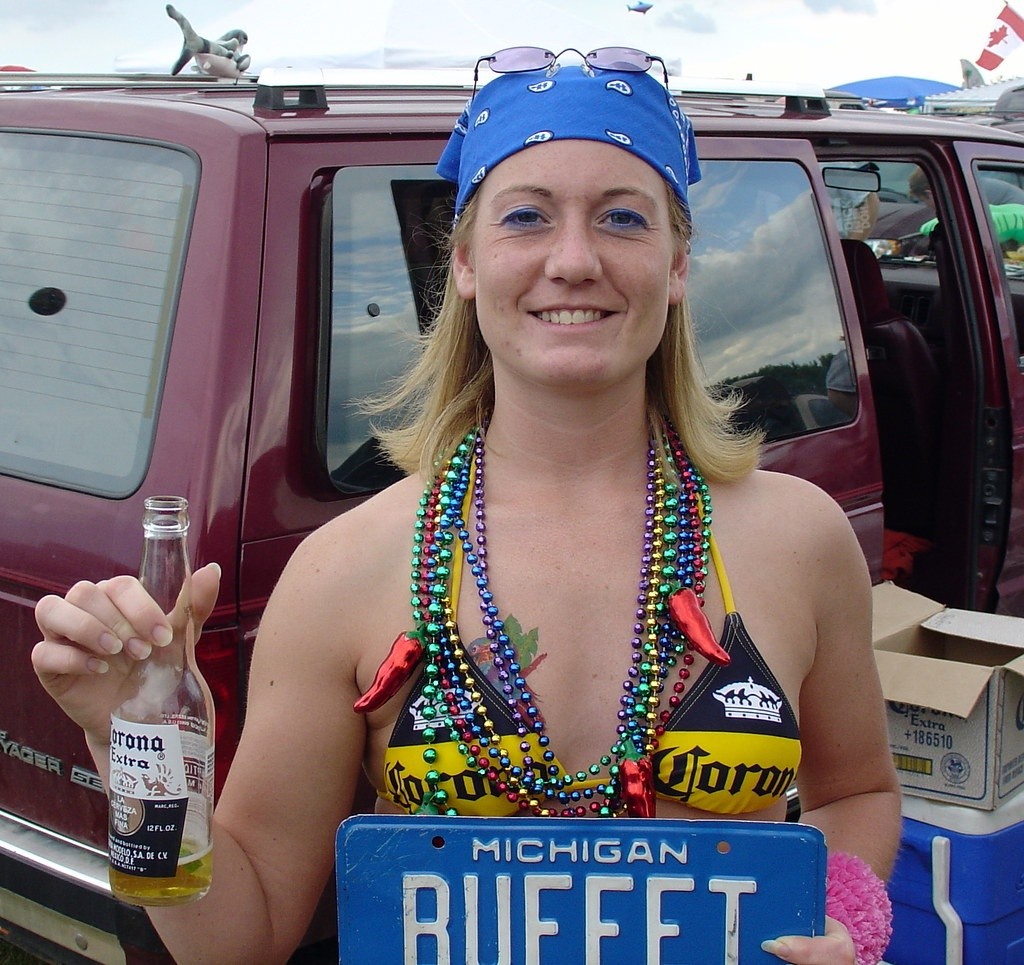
[469,47,670,104]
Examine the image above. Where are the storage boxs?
[882,796,1024,965]
[871,580,1024,812]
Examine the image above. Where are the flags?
[976,7,1024,70]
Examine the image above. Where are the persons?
[857,165,1024,250]
[29,44,904,965]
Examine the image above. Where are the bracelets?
[822,843,897,965]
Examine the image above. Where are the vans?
[0,60,1024,965]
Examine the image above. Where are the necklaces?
[355,403,732,818]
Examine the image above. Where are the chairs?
[840,237,943,537]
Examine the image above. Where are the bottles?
[107,493,213,903]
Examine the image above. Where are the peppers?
[619,740,656,818]
[668,582,731,666]
[353,623,425,711]
